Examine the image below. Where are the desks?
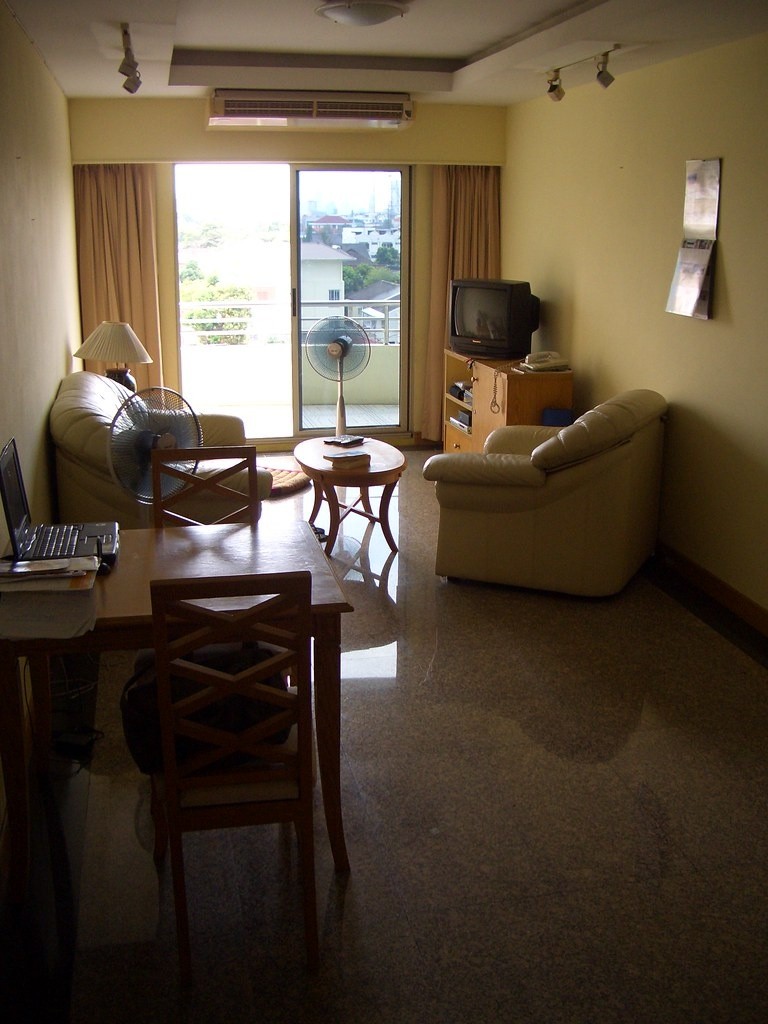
[0,518,355,901]
[294,435,406,557]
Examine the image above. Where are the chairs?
[135,446,290,681]
[146,570,321,979]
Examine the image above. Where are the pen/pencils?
[96,535,103,564]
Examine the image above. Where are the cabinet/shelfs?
[443,347,574,454]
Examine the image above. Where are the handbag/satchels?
[120,638,292,777]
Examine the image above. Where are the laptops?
[0,436,120,564]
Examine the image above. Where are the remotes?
[324,435,364,447]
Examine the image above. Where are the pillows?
[258,466,311,496]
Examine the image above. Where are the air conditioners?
[206,89,415,129]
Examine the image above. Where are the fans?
[107,386,204,528]
[305,315,372,435]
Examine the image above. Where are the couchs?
[423,389,669,599]
[49,370,273,530]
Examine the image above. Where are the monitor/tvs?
[448,279,540,361]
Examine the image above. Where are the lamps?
[314,0,409,27]
[547,69,565,102]
[596,53,616,88]
[73,321,153,393]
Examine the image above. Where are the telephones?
[520,350,569,372]
